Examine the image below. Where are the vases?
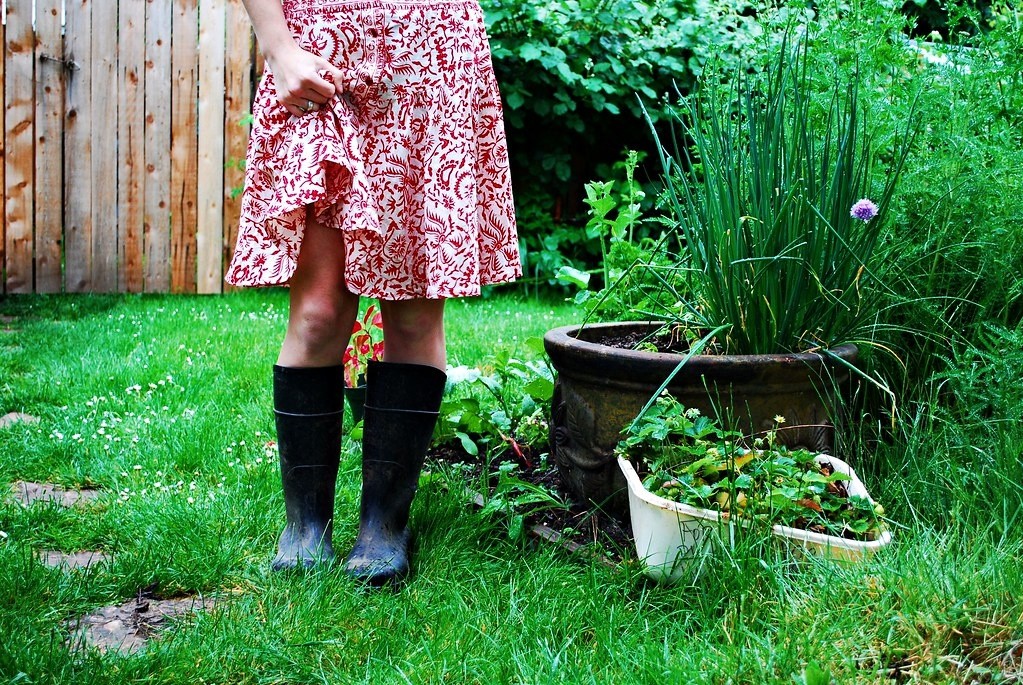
[345,373,368,424]
[541,315,875,527]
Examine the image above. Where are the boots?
[345,357,448,590]
[271,365,340,566]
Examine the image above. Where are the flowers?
[576,7,984,451]
[343,306,391,387]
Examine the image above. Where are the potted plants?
[619,320,895,590]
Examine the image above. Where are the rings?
[306,101,314,112]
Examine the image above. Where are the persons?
[226,0,523,592]
